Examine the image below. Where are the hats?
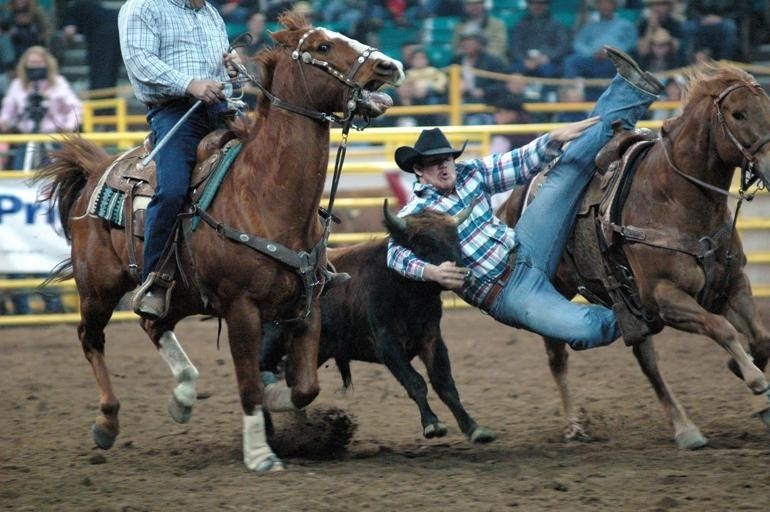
[395,128,468,173]
[459,22,488,46]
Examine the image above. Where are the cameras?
[27,68,46,80]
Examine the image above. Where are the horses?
[26,9,408,476]
[494,57,770,451]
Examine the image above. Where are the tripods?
[22,95,57,172]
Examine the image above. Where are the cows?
[258,196,498,445]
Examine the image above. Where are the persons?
[216,0,770,126]
[116,0,252,321]
[0,1,123,172]
[385,45,664,351]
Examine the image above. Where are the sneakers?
[137,284,165,319]
[602,43,665,96]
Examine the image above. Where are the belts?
[480,248,517,312]
